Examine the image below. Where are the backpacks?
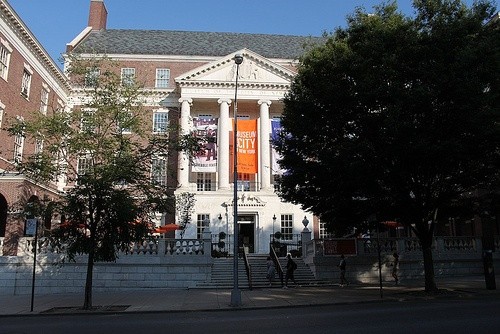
[291,260,298,270]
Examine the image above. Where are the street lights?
[226,53,244,310]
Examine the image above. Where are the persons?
[283,254,300,288]
[205,129,217,161]
[265,257,276,287]
[391,252,401,285]
[337,255,347,284]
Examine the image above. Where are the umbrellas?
[129,219,181,238]
[61,221,86,229]
